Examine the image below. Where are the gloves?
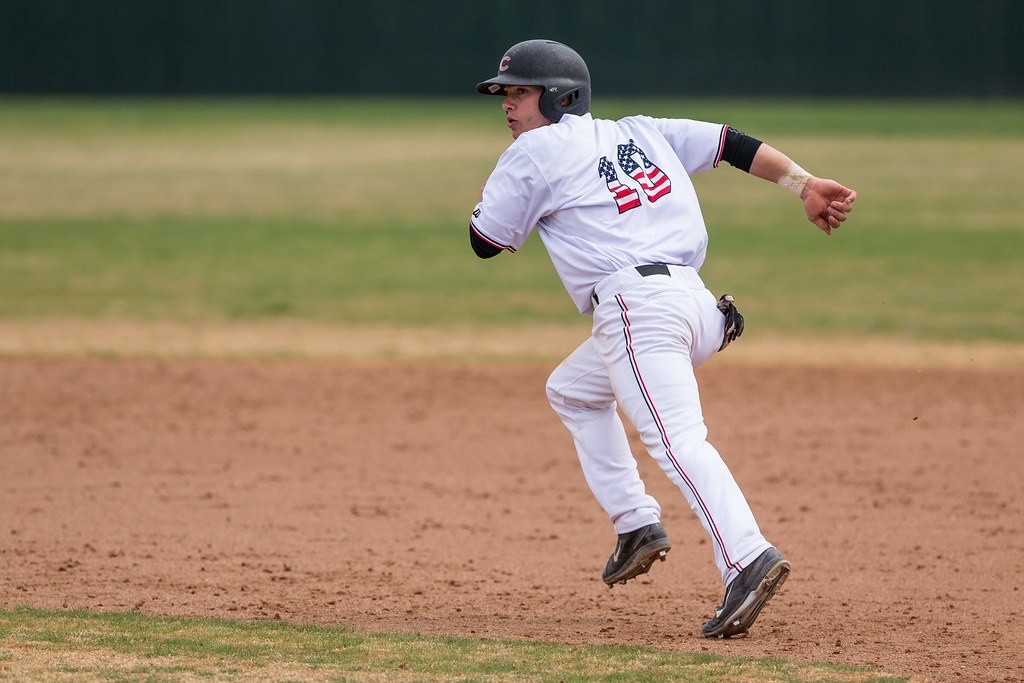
[717,294,745,351]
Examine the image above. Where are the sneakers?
[602,523,671,589]
[703,546,791,638]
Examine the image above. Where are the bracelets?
[777,162,811,197]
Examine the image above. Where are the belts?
[593,265,670,305]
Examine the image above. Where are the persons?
[469,39,856,636]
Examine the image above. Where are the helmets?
[476,39,591,122]
